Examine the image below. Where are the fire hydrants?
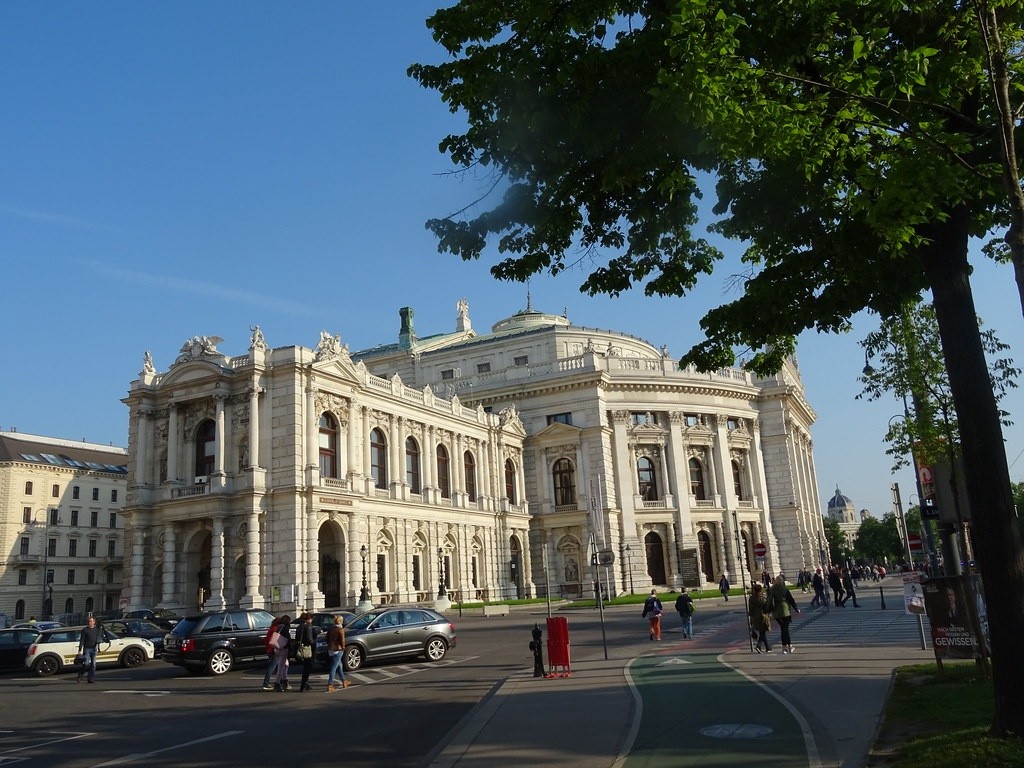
[529,622,546,677]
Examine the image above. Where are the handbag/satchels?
[269,625,288,649]
[752,631,758,639]
[74,653,85,666]
[765,587,776,613]
[687,597,694,616]
[297,643,312,658]
[721,589,726,593]
[653,599,660,614]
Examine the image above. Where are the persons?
[262,615,292,691]
[675,586,693,640]
[642,589,663,641]
[595,579,603,608]
[295,613,317,692]
[202,336,216,352]
[78,617,100,683]
[29,616,36,623]
[319,334,349,361]
[719,575,730,601]
[749,585,775,654]
[327,614,351,692]
[250,325,264,347]
[766,575,800,654]
[936,586,962,628]
[143,351,152,372]
[501,404,523,424]
[762,563,887,608]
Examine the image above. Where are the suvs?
[317,606,456,671]
[122,608,186,631]
[159,608,298,675]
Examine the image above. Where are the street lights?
[31,508,49,619]
[359,545,370,600]
[841,545,852,569]
[438,547,447,596]
[884,414,940,577]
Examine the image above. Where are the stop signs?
[754,543,766,556]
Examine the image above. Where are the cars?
[24,624,154,678]
[101,619,170,657]
[2,620,81,640]
[291,609,369,629]
[0,629,64,672]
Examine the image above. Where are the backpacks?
[646,598,653,611]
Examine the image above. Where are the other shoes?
[78,676,82,682]
[789,646,795,653]
[342,680,351,688]
[656,637,661,641]
[689,633,693,639]
[275,680,293,692]
[649,632,653,640]
[683,631,687,639]
[327,684,337,692]
[300,683,312,692]
[782,648,787,654]
[767,649,773,653]
[263,686,274,690]
[854,604,861,608]
[88,679,94,683]
[755,645,763,654]
